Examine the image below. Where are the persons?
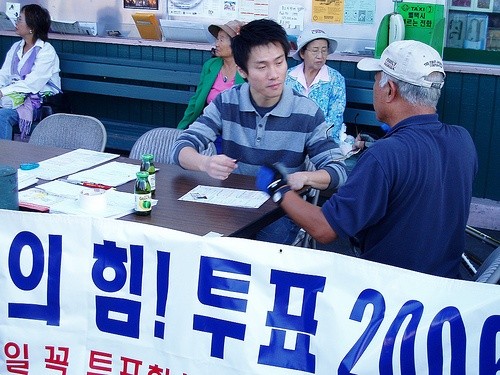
[0,4,61,140]
[176,19,246,130]
[255,40,479,280]
[285,28,347,140]
[172,19,348,245]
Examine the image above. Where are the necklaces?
[221,64,237,82]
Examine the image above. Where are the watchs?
[272,186,292,208]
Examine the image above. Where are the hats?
[356,39,446,89]
[292,28,339,61]
[207,20,241,39]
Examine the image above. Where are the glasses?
[15,16,24,23]
[325,112,365,162]
[303,47,331,55]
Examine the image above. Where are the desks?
[0,139,312,238]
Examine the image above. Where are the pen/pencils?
[235,155,243,164]
[77,182,116,190]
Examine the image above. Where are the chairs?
[129,127,217,168]
[28,113,107,152]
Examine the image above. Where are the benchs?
[55,51,385,171]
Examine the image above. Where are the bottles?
[133,171,151,216]
[140,153,156,199]
